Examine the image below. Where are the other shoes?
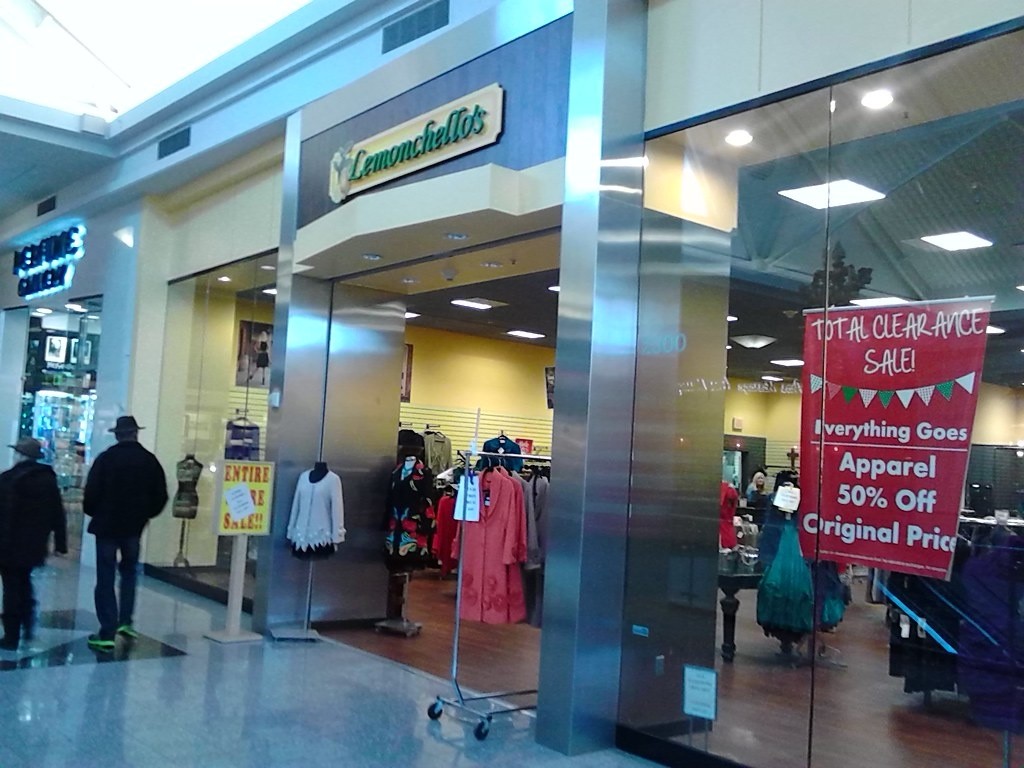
[0,637,19,651]
[21,629,34,640]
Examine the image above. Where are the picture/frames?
[400,344,414,403]
[235,319,274,390]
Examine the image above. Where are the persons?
[745,468,768,502]
[286,462,345,558]
[172,454,204,519]
[384,453,432,562]
[82,414,170,648]
[249,330,269,386]
[1,434,68,651]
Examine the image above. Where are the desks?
[718,550,762,664]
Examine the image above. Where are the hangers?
[420,425,451,443]
[228,408,261,429]
[445,454,551,493]
[484,429,519,447]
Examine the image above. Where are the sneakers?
[87,634,115,649]
[117,621,138,639]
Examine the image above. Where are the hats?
[107,416,145,432]
[7,437,45,458]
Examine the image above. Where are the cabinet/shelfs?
[963,444,1024,518]
